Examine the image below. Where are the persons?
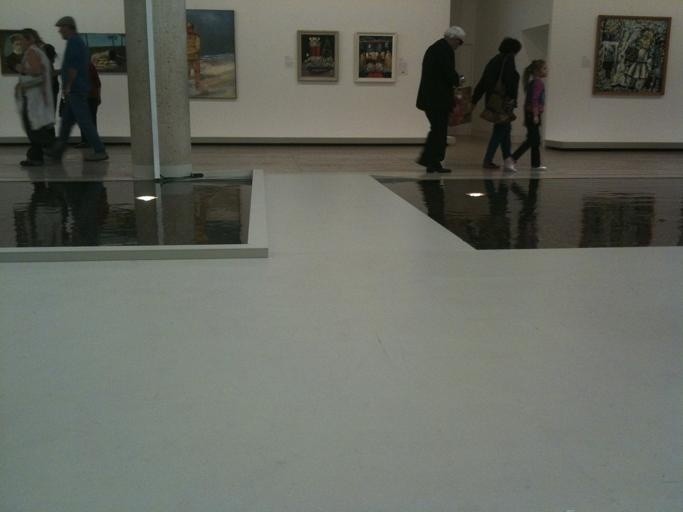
[13,16,109,167]
[511,58,549,172]
[5,36,24,69]
[470,37,523,170]
[413,26,466,175]
[357,40,391,78]
[185,19,203,94]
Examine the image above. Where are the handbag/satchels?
[449,86,472,126]
[480,85,516,123]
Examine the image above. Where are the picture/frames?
[294,27,342,85]
[351,30,398,86]
[591,14,673,99]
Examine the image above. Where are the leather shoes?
[417,157,451,173]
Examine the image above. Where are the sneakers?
[483,157,517,171]
[532,167,547,170]
[20,140,108,166]
